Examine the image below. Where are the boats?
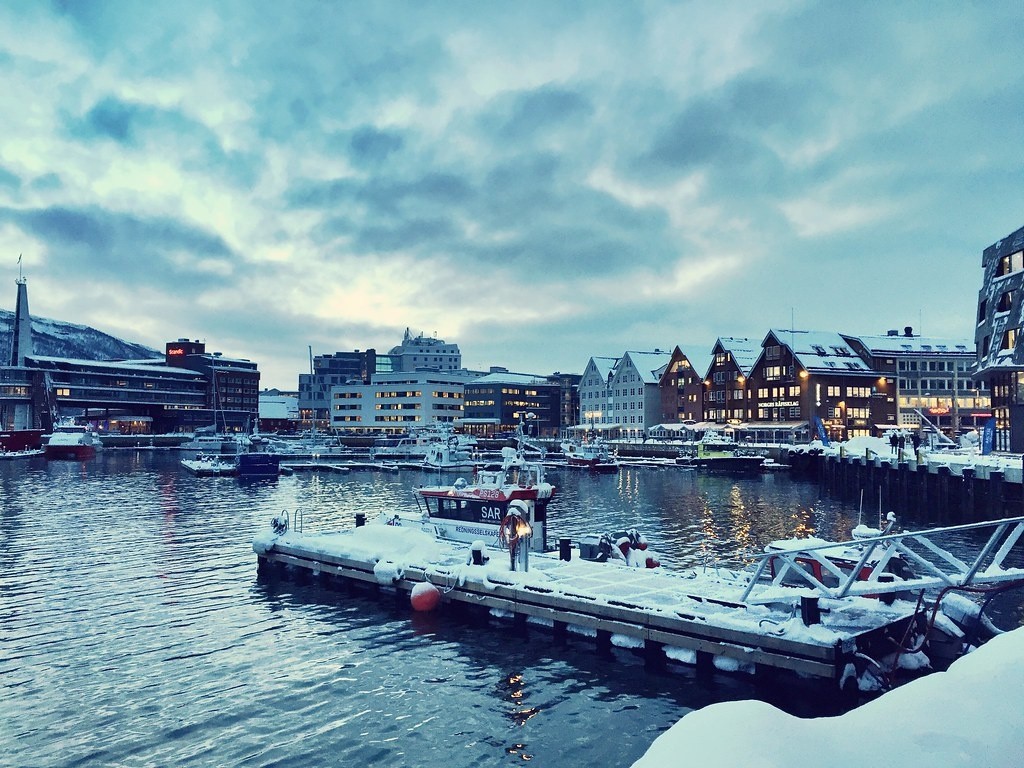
[378,447,555,554]
[765,486,913,605]
[43,425,95,459]
[91,432,103,453]
[676,428,791,473]
[181,432,479,459]
[560,406,619,471]
[423,445,474,473]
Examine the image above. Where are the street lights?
[970,389,981,451]
[925,392,934,451]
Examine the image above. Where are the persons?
[890,433,905,455]
[910,430,921,456]
